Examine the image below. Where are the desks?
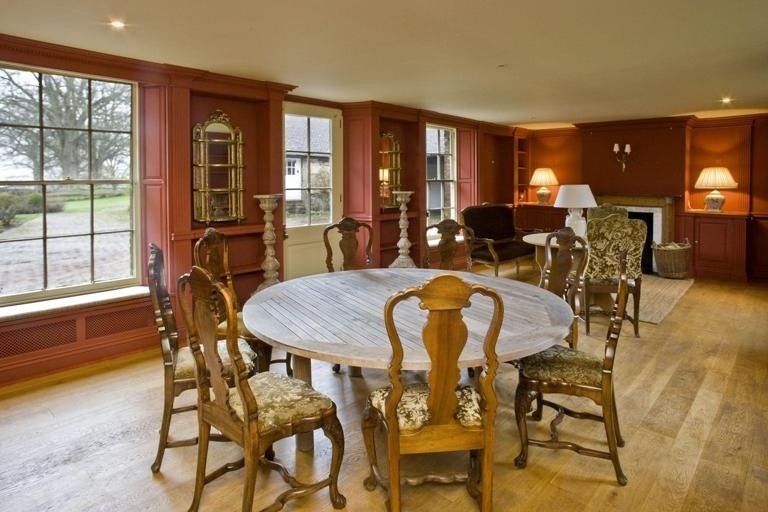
[521,231,590,290]
[242,265,575,453]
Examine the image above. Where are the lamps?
[694,166,739,212]
[553,185,599,237]
[529,166,560,207]
[613,143,632,173]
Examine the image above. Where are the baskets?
[651,238,691,278]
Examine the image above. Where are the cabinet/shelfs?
[672,214,749,284]
[377,127,404,213]
[194,111,246,227]
[749,217,768,285]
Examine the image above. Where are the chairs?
[460,203,544,277]
[423,217,476,270]
[363,273,506,512]
[575,212,649,339]
[587,203,629,221]
[513,247,629,487]
[537,226,590,353]
[322,217,375,376]
